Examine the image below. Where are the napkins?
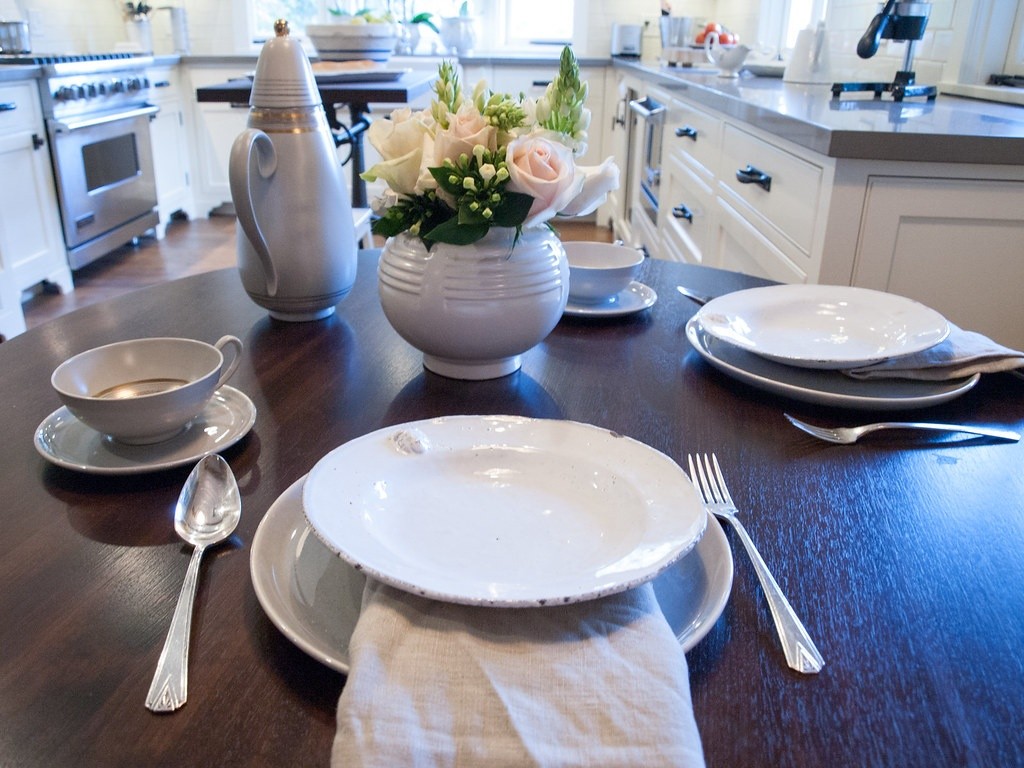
[846,321,1023,380]
[324,574,707,768]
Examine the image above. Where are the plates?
[34,384,258,474]
[695,283,953,370]
[563,280,657,316]
[300,412,709,608]
[684,312,981,411]
[247,472,743,684]
[744,60,785,76]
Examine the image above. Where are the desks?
[197,71,439,206]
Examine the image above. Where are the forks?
[782,412,1023,446]
[687,452,826,675]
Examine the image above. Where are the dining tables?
[0,246,1024,766]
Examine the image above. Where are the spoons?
[144,453,242,715]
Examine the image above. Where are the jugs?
[704,30,751,78]
[228,18,359,322]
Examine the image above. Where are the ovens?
[629,95,667,225]
[36,68,159,249]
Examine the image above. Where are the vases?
[374,225,569,380]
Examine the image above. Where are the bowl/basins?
[560,241,645,304]
[304,25,398,64]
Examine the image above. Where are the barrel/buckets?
[609,25,642,56]
[0,21,30,54]
[660,16,694,47]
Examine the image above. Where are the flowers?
[360,54,617,248]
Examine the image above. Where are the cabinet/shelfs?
[0,79,75,338]
[176,59,1024,349]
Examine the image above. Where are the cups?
[50,334,244,446]
